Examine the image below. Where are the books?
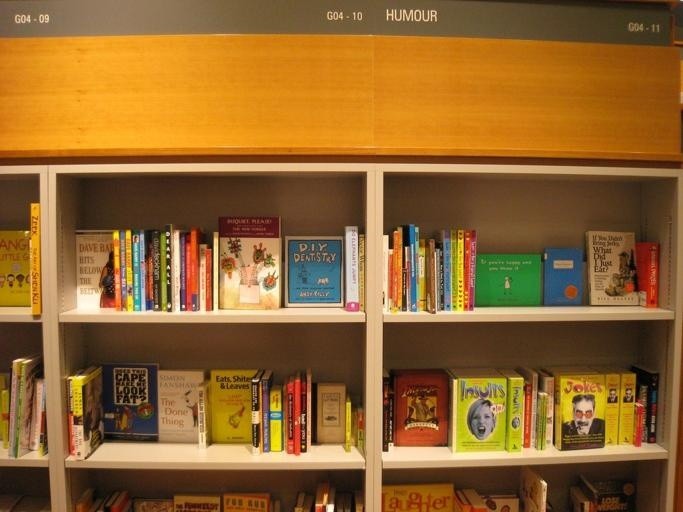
[382,465,637,511]
[383,224,658,308]
[382,365,660,452]
[0,352,48,459]
[75,218,365,312]
[1,202,42,315]
[66,360,364,461]
[76,475,363,512]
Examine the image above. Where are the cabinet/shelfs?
[0,162,682,512]
